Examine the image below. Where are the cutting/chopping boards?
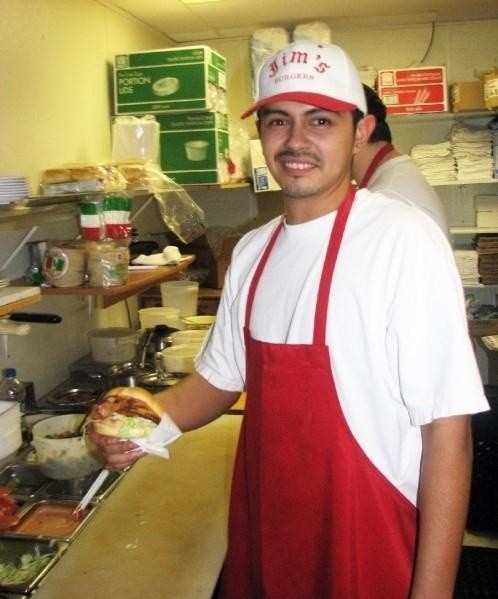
[0,285,43,318]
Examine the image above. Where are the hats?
[240,40,367,120]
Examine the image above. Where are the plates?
[47,386,100,407]
[0,277,10,289]
[0,176,33,205]
[135,371,191,391]
[150,77,180,97]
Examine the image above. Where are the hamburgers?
[90,386,165,440]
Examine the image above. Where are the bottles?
[0,368,28,439]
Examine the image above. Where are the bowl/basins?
[183,140,209,162]
[24,414,53,437]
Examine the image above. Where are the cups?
[254,166,268,190]
[84,236,131,288]
[44,241,84,289]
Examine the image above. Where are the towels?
[131,245,185,266]
[410,120,497,182]
[452,235,497,286]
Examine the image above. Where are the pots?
[136,307,180,331]
[154,315,216,374]
[32,414,107,480]
[87,326,140,364]
[105,330,155,390]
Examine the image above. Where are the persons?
[88,40,490,596]
[352,82,451,253]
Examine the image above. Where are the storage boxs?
[113,45,226,115]
[160,114,230,185]
[449,81,486,112]
[250,140,282,194]
[483,71,498,110]
[377,66,447,113]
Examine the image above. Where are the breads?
[42,161,147,191]
